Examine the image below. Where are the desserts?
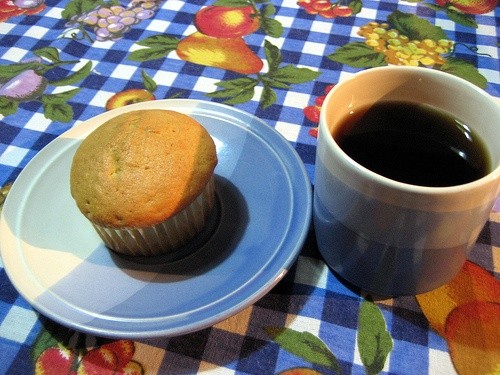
[69,109,218,256]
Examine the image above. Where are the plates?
[1,98,313,339]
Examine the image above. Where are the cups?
[312,66,500,295]
